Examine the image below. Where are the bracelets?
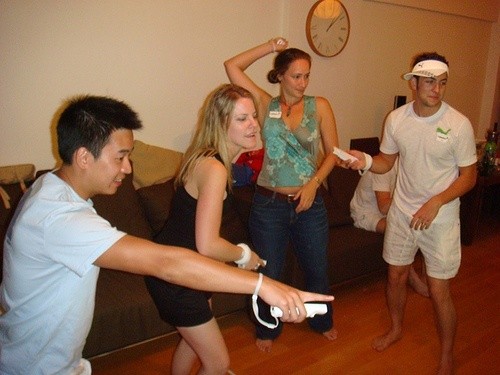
[311,174,324,188]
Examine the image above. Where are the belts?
[256,185,327,204]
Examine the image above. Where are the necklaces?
[279,94,304,117]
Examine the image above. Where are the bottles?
[483,142,497,169]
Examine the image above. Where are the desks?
[459,165,499,246]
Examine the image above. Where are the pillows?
[141,180,250,243]
[36,169,154,279]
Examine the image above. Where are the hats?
[403,60,450,80]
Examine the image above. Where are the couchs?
[0,168,293,359]
[325,166,392,285]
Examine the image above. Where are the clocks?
[306,1,350,57]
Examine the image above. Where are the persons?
[224,36,339,353]
[145,83,265,374]
[348,137,445,297]
[336,52,479,375]
[0,96,336,375]
